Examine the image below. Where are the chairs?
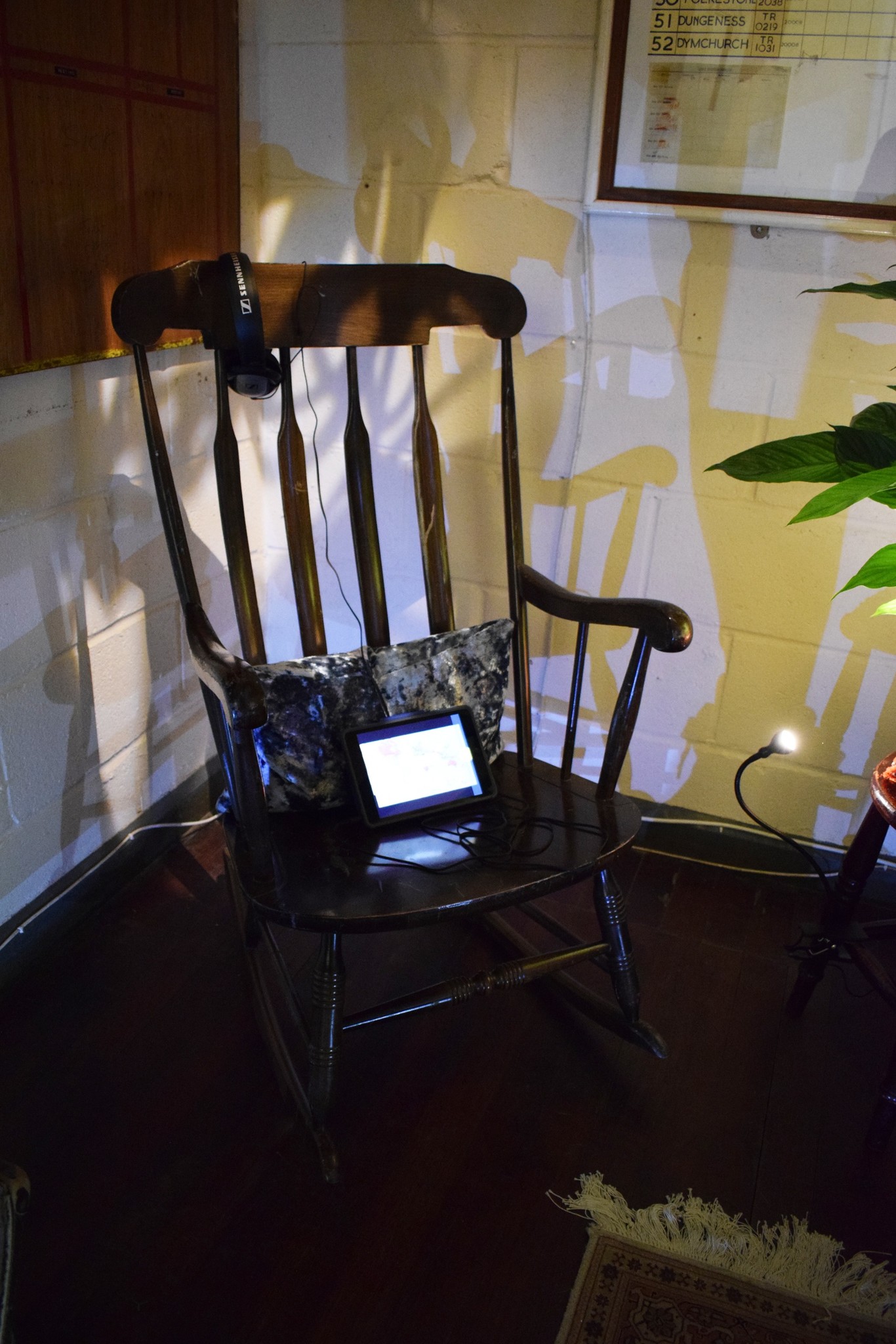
[112,264,696,1226]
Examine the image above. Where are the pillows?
[216,621,523,831]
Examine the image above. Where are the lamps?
[735,729,855,963]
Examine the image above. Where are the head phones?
[220,251,282,400]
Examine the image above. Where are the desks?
[790,756,896,1018]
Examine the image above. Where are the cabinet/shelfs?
[0,0,240,377]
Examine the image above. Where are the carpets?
[558,1160,896,1344]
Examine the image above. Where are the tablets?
[342,705,497,826]
